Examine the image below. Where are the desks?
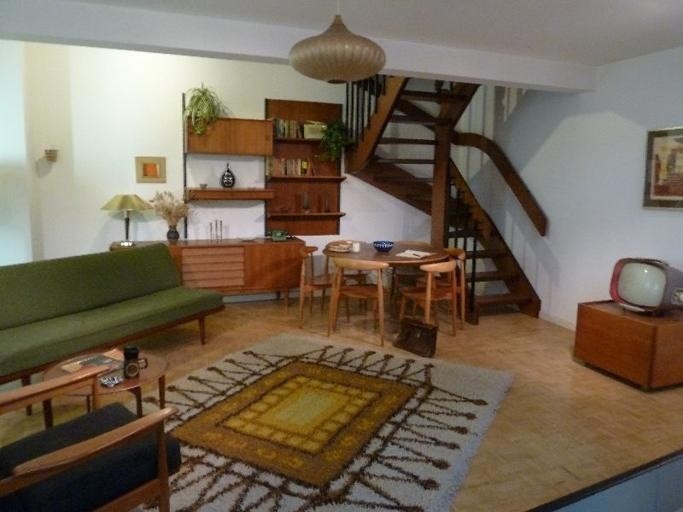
[163,235,305,311]
[43,344,170,432]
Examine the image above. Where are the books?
[271,117,303,139]
[268,157,317,179]
[396,248,438,259]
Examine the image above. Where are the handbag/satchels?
[392,318,438,358]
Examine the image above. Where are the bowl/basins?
[373,240,393,252]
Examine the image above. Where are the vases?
[166,225,180,242]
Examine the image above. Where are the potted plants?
[185,86,222,137]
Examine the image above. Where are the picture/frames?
[641,126,683,209]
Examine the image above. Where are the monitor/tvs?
[609,258,683,316]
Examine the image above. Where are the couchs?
[0,241,226,418]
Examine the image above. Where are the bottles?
[122,343,148,378]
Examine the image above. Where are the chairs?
[297,236,468,347]
[0,372,183,512]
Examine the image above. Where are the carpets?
[120,330,514,512]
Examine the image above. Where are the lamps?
[100,194,154,248]
[284,1,384,86]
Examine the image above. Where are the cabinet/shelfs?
[264,98,347,237]
[184,118,274,202]
[569,300,682,390]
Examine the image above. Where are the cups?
[352,242,360,253]
